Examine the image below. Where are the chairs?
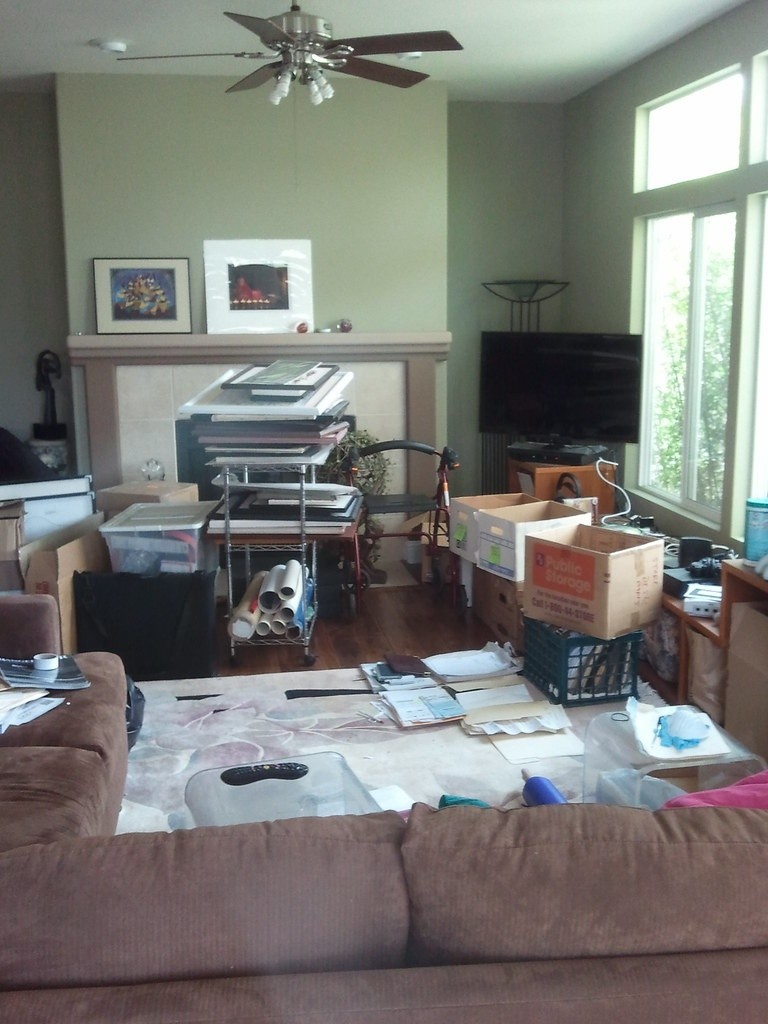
[341,441,460,613]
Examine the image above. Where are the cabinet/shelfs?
[508,458,768,759]
[209,460,321,666]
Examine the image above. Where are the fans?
[117,0,464,105]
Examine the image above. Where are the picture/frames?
[177,360,355,535]
[93,257,192,334]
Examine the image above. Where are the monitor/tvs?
[479,332,644,450]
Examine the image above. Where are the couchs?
[0,591,768,1024]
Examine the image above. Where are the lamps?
[268,60,335,107]
[481,280,570,332]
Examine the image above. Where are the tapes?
[32,653,59,671]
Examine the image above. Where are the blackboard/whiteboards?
[0,475,98,545]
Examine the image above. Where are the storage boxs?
[0,478,666,709]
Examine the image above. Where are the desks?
[583,705,768,805]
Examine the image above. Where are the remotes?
[220,763,309,786]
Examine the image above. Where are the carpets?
[114,653,672,839]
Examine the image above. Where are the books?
[0,655,91,690]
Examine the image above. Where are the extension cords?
[604,522,650,536]
